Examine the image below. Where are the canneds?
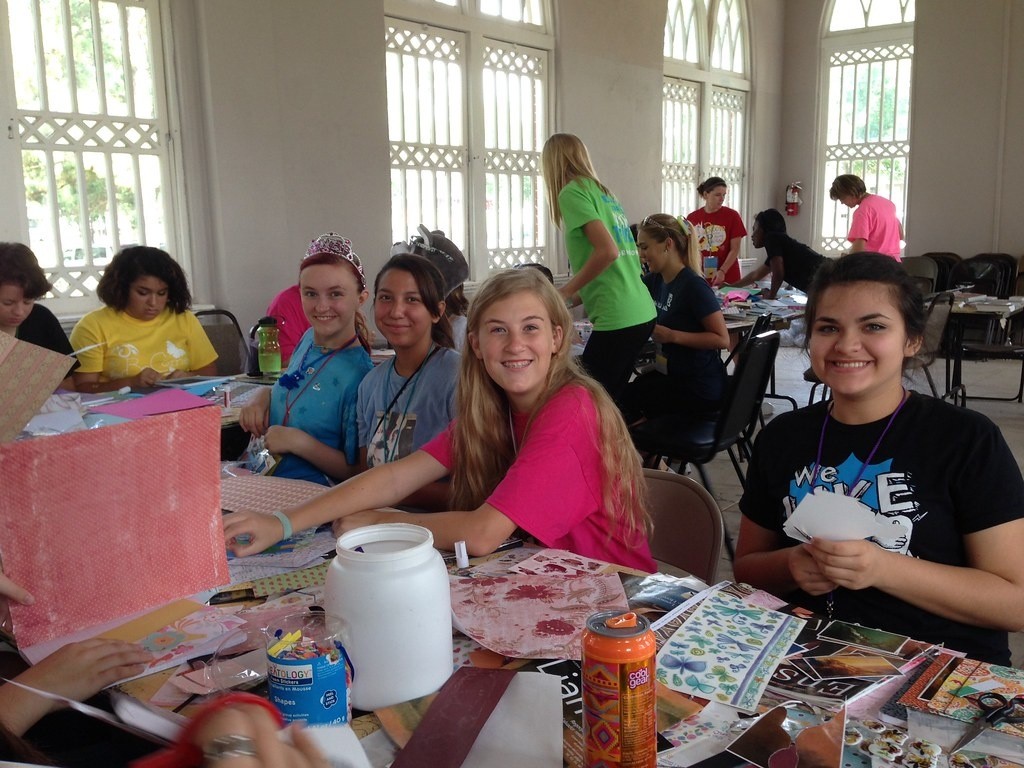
[580,610,657,768]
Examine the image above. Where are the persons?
[722,208,834,300]
[0,569,154,736]
[626,214,735,477]
[0,241,81,391]
[68,246,219,394]
[267,286,312,367]
[540,132,658,406]
[192,701,329,768]
[356,222,470,512]
[222,266,659,576]
[685,177,747,289]
[830,174,904,262]
[734,251,1024,667]
[238,234,376,488]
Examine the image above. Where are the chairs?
[195,251,1024,588]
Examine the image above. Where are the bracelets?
[718,269,726,275]
[273,511,292,542]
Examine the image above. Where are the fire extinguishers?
[785,181,803,216]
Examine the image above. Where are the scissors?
[949,692,1024,756]
[130,691,287,768]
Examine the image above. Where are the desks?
[27,289,812,454]
[926,289,1024,408]
[0,462,1024,768]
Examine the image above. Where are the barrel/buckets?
[260,612,352,730]
[325,523,452,711]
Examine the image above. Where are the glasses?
[642,216,671,238]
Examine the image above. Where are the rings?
[202,733,257,764]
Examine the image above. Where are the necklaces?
[278,335,359,427]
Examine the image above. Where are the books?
[766,620,1024,766]
[945,291,1015,311]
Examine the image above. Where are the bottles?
[257,316,281,372]
